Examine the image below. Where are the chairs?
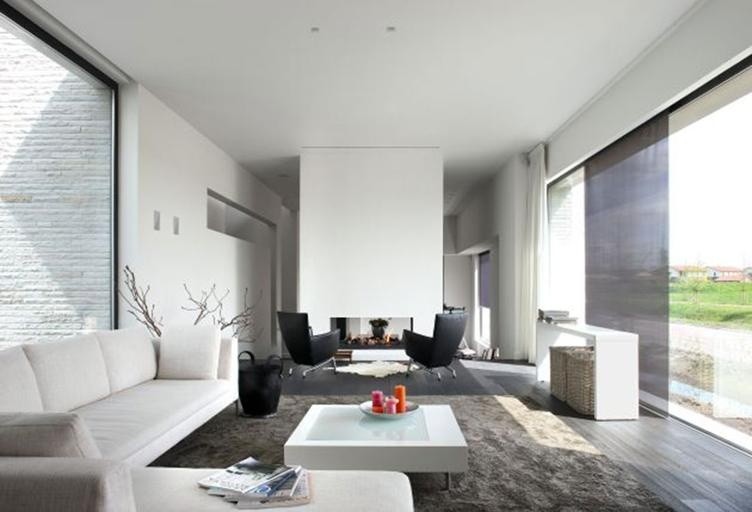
[402,312,468,381]
[276,311,341,379]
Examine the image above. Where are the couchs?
[0,323,415,512]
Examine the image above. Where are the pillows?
[157,324,220,380]
[1,412,101,458]
[0,346,43,412]
[22,334,111,412]
[95,326,158,394]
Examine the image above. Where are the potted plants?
[369,319,388,339]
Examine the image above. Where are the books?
[199,456,310,509]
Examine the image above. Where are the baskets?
[549,346,596,417]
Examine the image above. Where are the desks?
[535,320,640,422]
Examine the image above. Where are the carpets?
[147,393,676,512]
[322,361,422,379]
[336,361,421,378]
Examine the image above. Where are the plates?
[358,401,420,418]
